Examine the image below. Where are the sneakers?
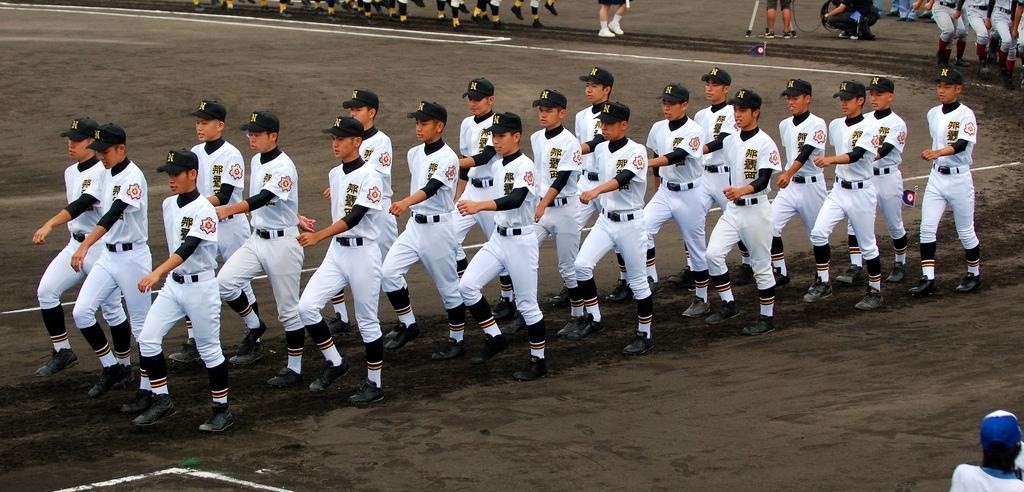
[647,276,664,295]
[704,301,740,324]
[493,21,509,30]
[347,382,384,405]
[282,12,292,17]
[412,0,425,7]
[850,36,858,40]
[557,313,584,336]
[432,337,464,359]
[513,355,548,380]
[731,263,753,286]
[622,331,654,356]
[308,357,351,392]
[886,12,898,16]
[88,363,126,398]
[327,312,352,336]
[956,59,970,67]
[491,296,513,318]
[34,348,79,376]
[120,389,152,411]
[764,28,774,39]
[532,19,544,29]
[459,4,469,14]
[553,287,568,302]
[606,279,631,303]
[267,367,304,385]
[469,333,508,364]
[384,322,420,349]
[782,32,792,39]
[886,262,906,282]
[608,21,624,35]
[400,20,414,30]
[122,364,134,390]
[568,313,605,341]
[199,403,234,431]
[237,319,266,356]
[804,281,832,302]
[598,28,615,38]
[896,18,906,21]
[909,275,936,296]
[906,18,912,22]
[544,2,557,16]
[956,272,979,293]
[229,342,264,364]
[773,267,790,286]
[327,15,340,23]
[667,266,693,286]
[386,325,402,338]
[839,31,849,38]
[471,14,481,23]
[453,24,464,32]
[682,295,710,318]
[168,338,200,363]
[836,264,865,283]
[511,5,523,20]
[742,314,775,335]
[130,394,177,426]
[855,286,884,309]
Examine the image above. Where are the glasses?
[246,132,260,138]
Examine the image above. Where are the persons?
[194,1,631,36]
[30,67,981,429]
[760,1,1023,90]
[949,409,1024,492]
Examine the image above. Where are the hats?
[594,102,630,123]
[192,101,225,122]
[343,90,378,112]
[727,89,761,109]
[240,111,279,132]
[782,79,811,96]
[980,410,1023,449]
[865,77,894,93]
[407,101,447,122]
[86,123,126,152]
[702,68,731,85]
[579,67,613,86]
[463,78,493,98]
[486,112,521,133]
[656,84,689,102]
[158,148,198,173]
[935,68,963,83]
[533,90,566,109]
[60,116,99,141]
[833,80,866,98]
[320,116,364,137]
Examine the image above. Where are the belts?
[940,2,955,8]
[836,177,863,189]
[974,6,987,10]
[661,178,693,191]
[541,198,567,207]
[603,209,633,221]
[472,178,493,187]
[792,177,816,183]
[583,170,598,181]
[256,229,283,239]
[173,272,198,284]
[412,212,439,223]
[336,238,362,246]
[733,198,757,206]
[73,233,85,242]
[106,243,132,252]
[1000,7,1011,13]
[497,227,521,236]
[874,168,889,175]
[934,164,959,174]
[705,166,728,173]
[220,215,233,222]
